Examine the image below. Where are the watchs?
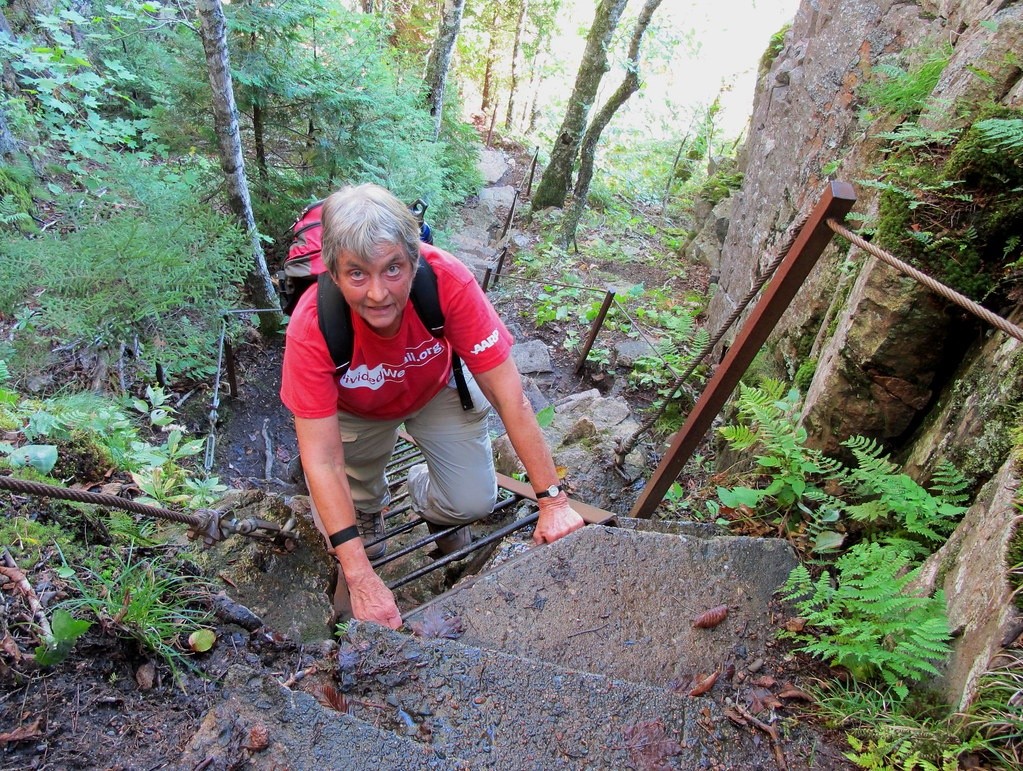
[536,485,563,499]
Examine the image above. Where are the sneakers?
[354,506,386,559]
[426,520,471,562]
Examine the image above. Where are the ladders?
[326,425,616,635]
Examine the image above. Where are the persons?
[281,183,584,631]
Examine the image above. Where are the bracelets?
[329,525,359,548]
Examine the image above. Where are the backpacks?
[277,198,444,376]
